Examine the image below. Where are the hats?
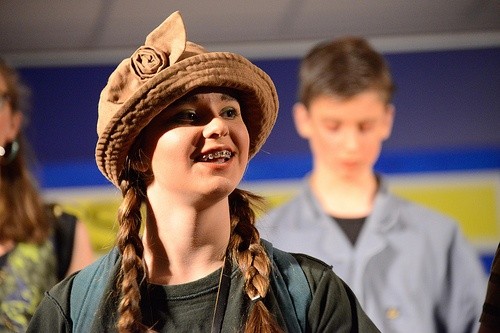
[95,10,279,190]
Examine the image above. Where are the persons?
[477,242,500,333]
[26,11,381,333]
[253,34,487,333]
[0,59,93,333]
